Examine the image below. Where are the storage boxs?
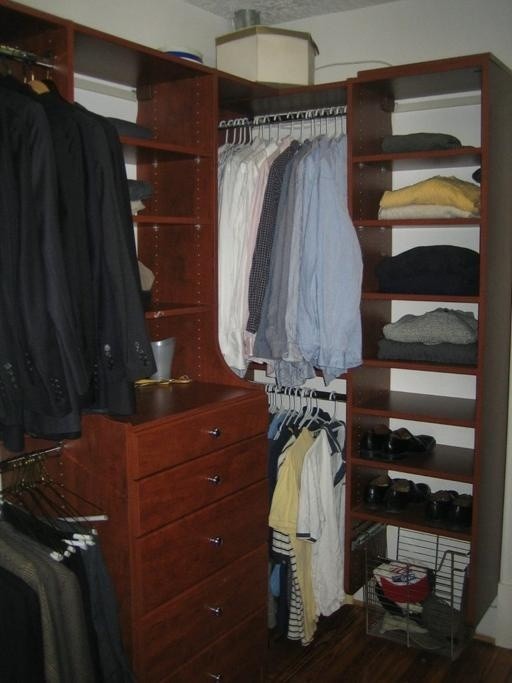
[214,24,320,90]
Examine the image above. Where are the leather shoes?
[363,475,402,512]
[423,490,457,527]
[379,428,436,464]
[384,478,431,514]
[359,424,395,460]
[446,493,473,533]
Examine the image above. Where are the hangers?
[262,382,346,452]
[1,443,109,564]
[220,103,348,154]
[0,45,65,97]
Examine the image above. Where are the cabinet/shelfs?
[32,377,266,682]
[74,25,218,382]
[346,51,511,628]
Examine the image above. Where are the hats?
[379,611,450,650]
[372,557,433,604]
